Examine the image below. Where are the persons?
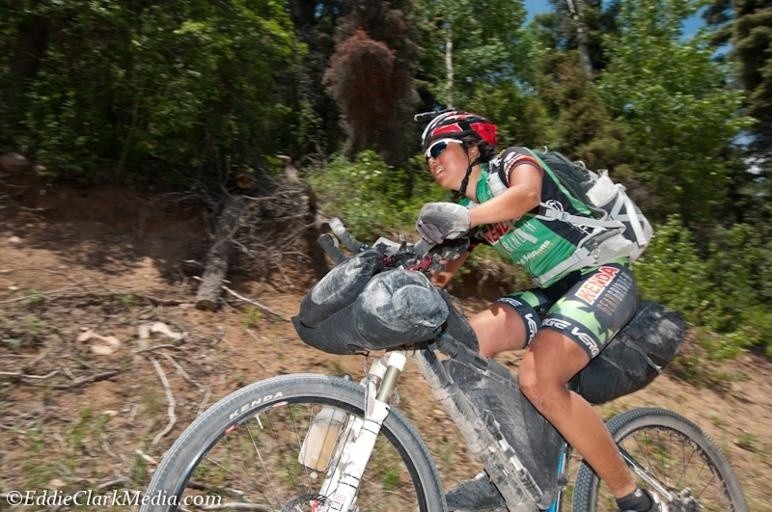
[415,109,657,512]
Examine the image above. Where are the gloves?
[415,201,471,244]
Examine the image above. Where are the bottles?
[296,373,354,474]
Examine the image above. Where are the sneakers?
[443,469,508,511]
[623,486,669,512]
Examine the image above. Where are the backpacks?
[498,146,653,287]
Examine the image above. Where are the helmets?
[419,109,498,157]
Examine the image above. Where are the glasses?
[424,138,464,165]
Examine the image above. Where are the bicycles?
[136,211,750,512]
